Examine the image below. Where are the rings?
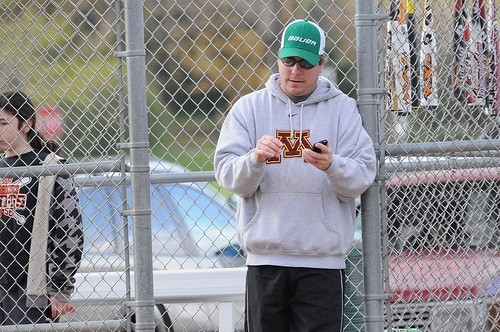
[314,159,319,164]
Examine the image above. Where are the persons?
[213,18,377,332]
[0,91,84,331]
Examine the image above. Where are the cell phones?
[311,140,328,153]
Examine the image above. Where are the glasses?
[279,57,322,69]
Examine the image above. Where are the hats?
[278,18,325,67]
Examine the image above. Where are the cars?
[48,139,498,331]
